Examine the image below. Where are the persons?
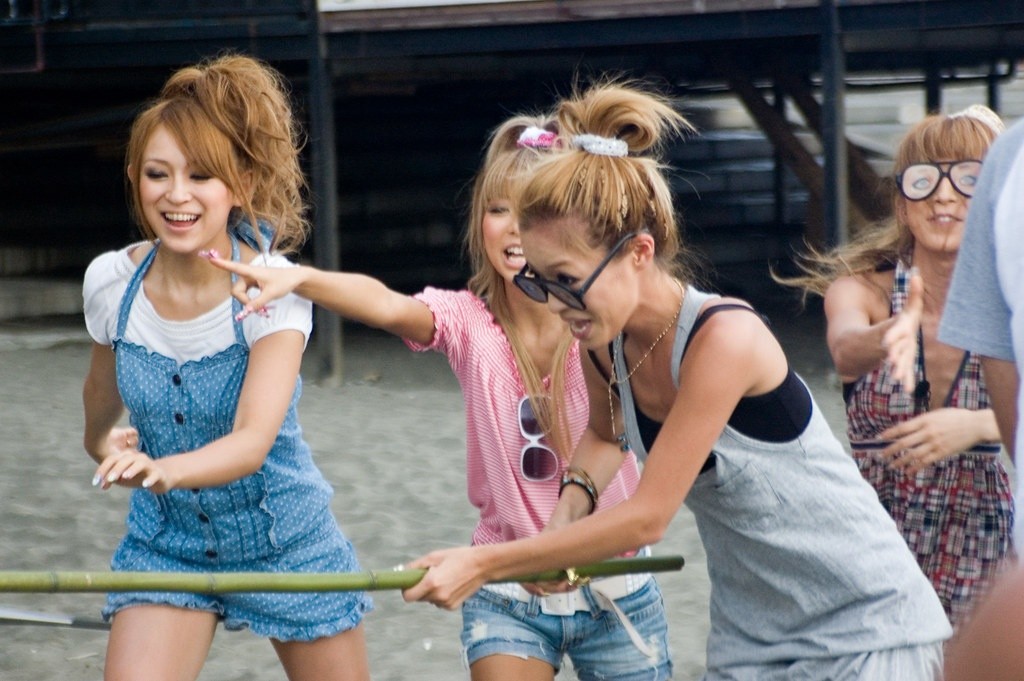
[776,105,1015,634]
[81,54,370,681]
[393,83,953,680]
[934,119,1024,567]
[209,114,672,681]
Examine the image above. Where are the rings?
[126,440,130,447]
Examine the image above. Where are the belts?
[482,569,652,658]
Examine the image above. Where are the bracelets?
[559,468,598,514]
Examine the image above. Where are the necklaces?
[608,281,705,434]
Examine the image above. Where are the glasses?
[895,160,983,202]
[512,229,650,312]
[517,394,558,483]
